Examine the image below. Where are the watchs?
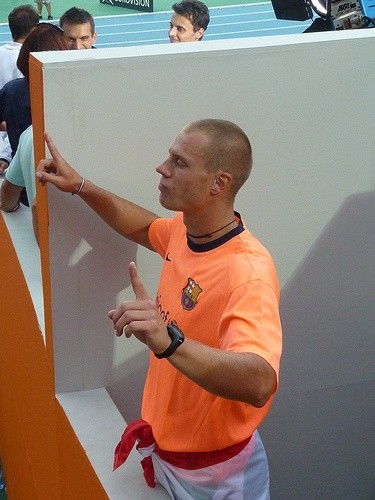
[154,320,184,359]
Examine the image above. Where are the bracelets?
[72,177,84,195]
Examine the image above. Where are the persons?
[60,6,97,50]
[0,23,66,249]
[0,5,39,89]
[37,118,282,500]
[34,0,53,19]
[169,0,209,43]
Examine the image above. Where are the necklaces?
[186,218,236,238]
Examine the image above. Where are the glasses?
[37,22,64,35]
